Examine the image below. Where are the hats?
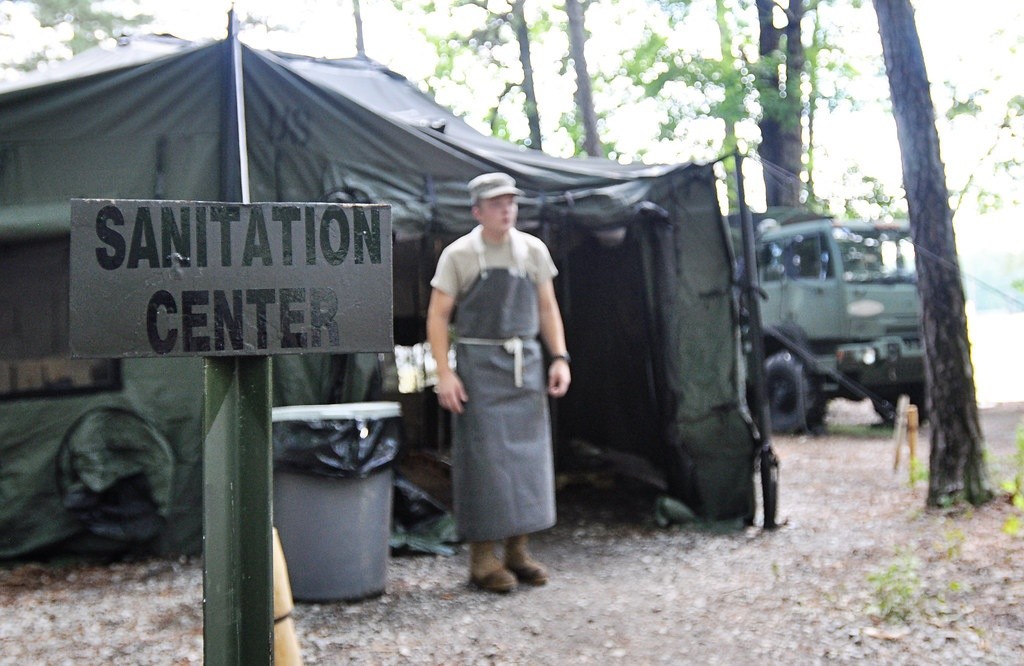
[468,171,523,206]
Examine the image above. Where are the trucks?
[723,210,937,436]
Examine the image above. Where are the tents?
[0,41,777,570]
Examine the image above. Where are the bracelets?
[550,355,570,361]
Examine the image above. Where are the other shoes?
[469,567,519,591]
[503,557,549,585]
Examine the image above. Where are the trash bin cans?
[272,402,404,600]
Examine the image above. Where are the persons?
[425,172,571,592]
